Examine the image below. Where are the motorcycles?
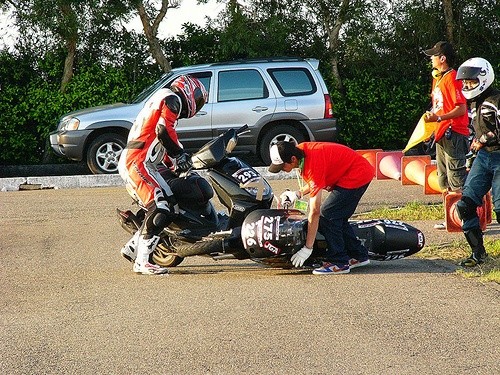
[175,189,425,272]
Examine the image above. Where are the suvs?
[49,56,339,176]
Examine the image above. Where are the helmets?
[455,57,495,101]
[170,74,207,118]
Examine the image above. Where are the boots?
[121,231,168,275]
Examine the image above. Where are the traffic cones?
[444,193,488,232]
[375,151,405,181]
[486,191,492,225]
[355,149,382,179]
[401,155,432,187]
[423,164,449,195]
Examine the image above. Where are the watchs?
[437,116,442,122]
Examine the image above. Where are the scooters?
[117,123,274,268]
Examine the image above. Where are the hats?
[268,141,293,173]
[422,41,455,56]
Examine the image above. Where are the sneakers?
[434,222,446,230]
[349,258,371,270]
[462,250,487,266]
[312,262,350,275]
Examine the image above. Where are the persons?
[267,137,375,274]
[452,57,500,267]
[422,42,470,228]
[118,74,209,275]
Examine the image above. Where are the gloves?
[280,191,297,205]
[290,245,313,268]
[176,153,192,169]
